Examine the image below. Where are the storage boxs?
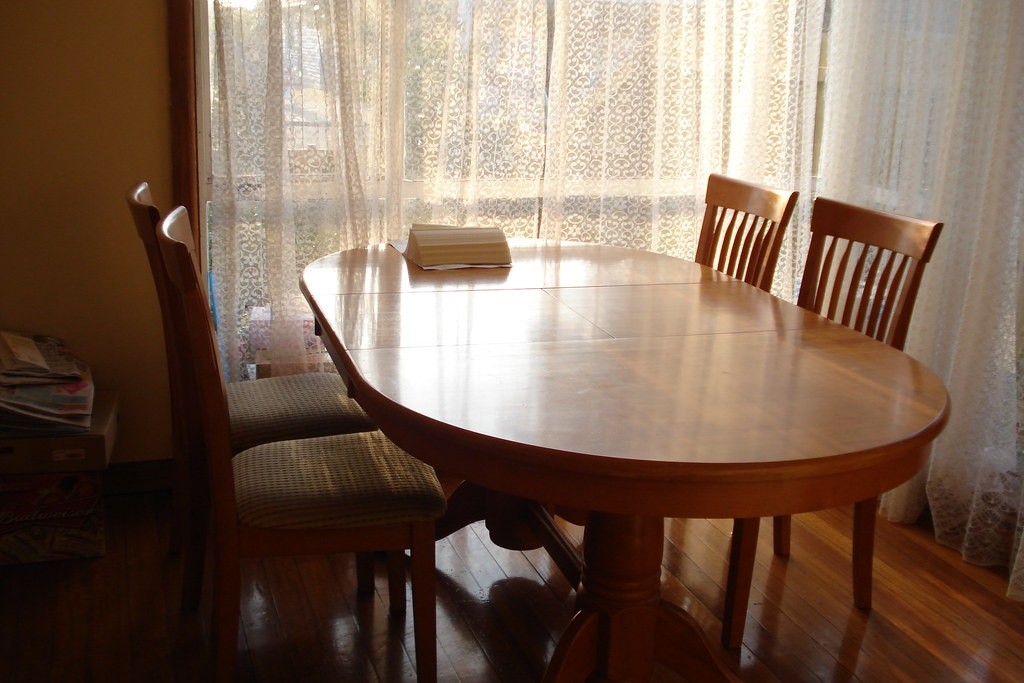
[0,386,121,567]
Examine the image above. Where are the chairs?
[156,203,445,683]
[694,174,799,296]
[721,197,947,647]
[126,182,408,635]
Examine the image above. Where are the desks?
[297,238,953,683]
